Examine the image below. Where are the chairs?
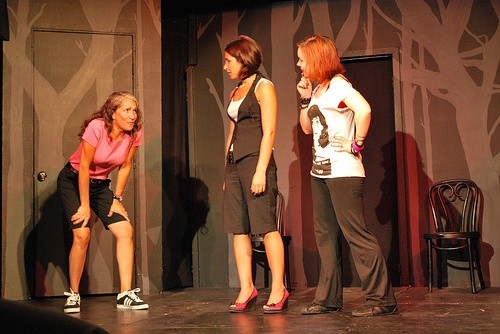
[423,178,486,294]
[251,192,292,291]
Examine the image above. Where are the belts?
[66,162,111,184]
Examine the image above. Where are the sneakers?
[116,287,150,309]
[63,288,80,313]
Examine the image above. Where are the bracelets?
[300,97,311,109]
[351,141,364,156]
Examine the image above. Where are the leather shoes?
[301,302,343,315]
[352,304,398,317]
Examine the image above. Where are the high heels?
[228,287,259,312]
[263,288,289,313]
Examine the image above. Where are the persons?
[57,91,148,313]
[223,37,289,312]
[296,34,398,314]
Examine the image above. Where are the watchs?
[113,195,123,201]
